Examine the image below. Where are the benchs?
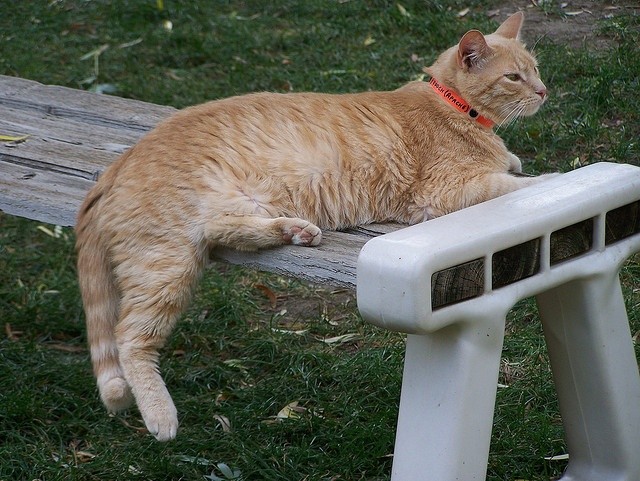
[0,73,639,481]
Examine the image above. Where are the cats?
[73,9,549,445]
